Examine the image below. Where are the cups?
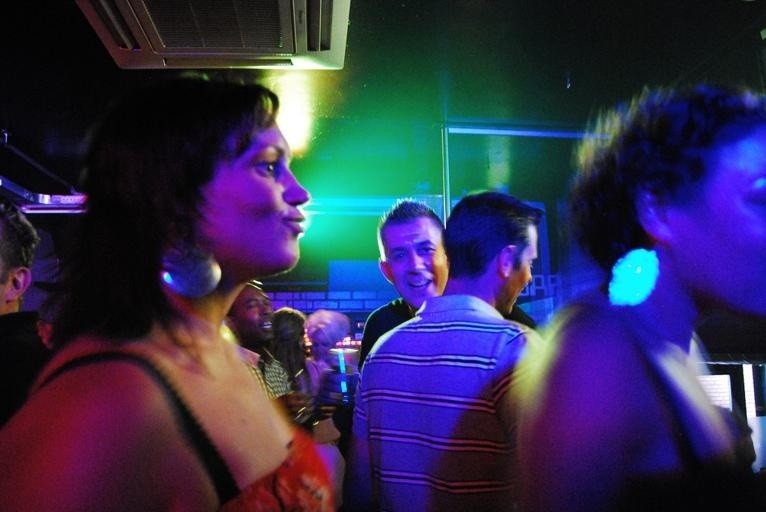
[327,348,359,406]
